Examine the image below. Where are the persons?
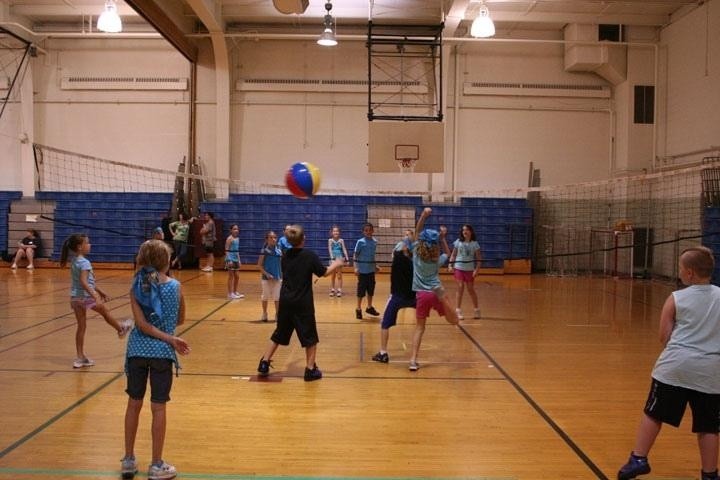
[372,233,419,364]
[616,244,719,480]
[255,223,483,322]
[11,227,43,270]
[152,227,165,242]
[224,224,246,300]
[168,211,199,270]
[257,224,348,381]
[58,231,133,368]
[121,238,192,480]
[199,211,219,273]
[408,206,460,372]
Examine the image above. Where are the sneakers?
[202,266,214,272]
[228,292,244,298]
[372,352,388,362]
[304,362,322,381]
[435,286,445,303]
[122,459,137,475]
[262,315,267,321]
[618,453,651,480]
[73,358,95,368]
[148,462,176,480]
[329,288,343,297]
[27,264,34,269]
[12,264,17,269]
[409,362,420,370]
[474,311,481,319]
[118,319,131,336]
[456,310,463,320]
[258,356,269,373]
[366,307,379,316]
[356,309,362,319]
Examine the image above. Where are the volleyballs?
[285,161,320,198]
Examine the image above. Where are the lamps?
[96,0,122,32]
[317,0,338,46]
[471,0,495,38]
